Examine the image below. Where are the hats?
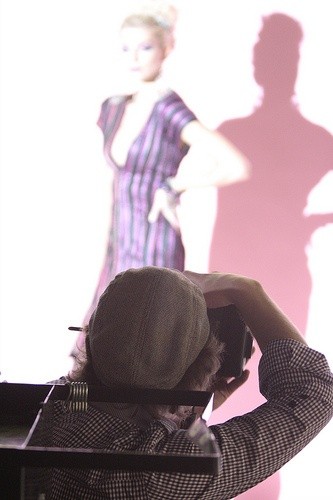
[88,266,210,391]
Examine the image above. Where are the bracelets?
[160,183,180,198]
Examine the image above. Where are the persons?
[22,264,333,500]
[70,13,255,364]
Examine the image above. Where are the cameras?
[207,304,252,378]
